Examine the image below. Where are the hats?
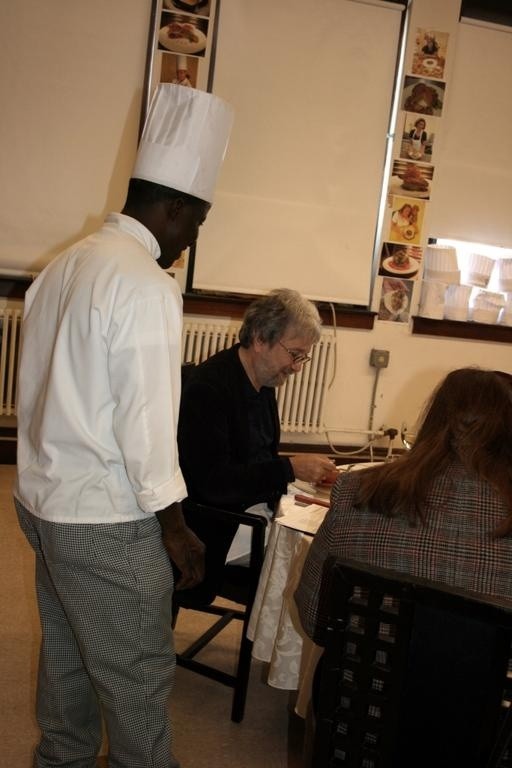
[419,244,512,327]
[129,83,237,208]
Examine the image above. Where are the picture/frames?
[137,0,221,298]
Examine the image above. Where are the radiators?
[0,310,338,434]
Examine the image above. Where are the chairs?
[174,362,273,725]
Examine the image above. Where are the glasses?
[279,340,312,366]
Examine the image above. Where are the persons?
[170,70,193,89]
[384,278,410,293]
[11,178,212,768]
[293,367,511,768]
[166,286,343,609]
[391,204,411,230]
[406,118,428,161]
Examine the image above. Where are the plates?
[400,81,446,119]
[384,290,409,315]
[389,174,433,199]
[382,254,419,275]
